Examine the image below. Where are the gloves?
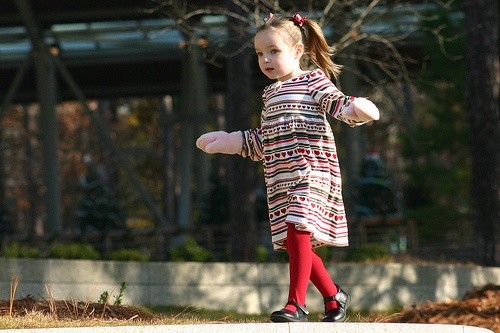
[196,130,242,156]
[341,97,381,124]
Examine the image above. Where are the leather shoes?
[323,284,351,322]
[270,301,309,323]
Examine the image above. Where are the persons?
[196,11,381,322]
[76,149,128,247]
